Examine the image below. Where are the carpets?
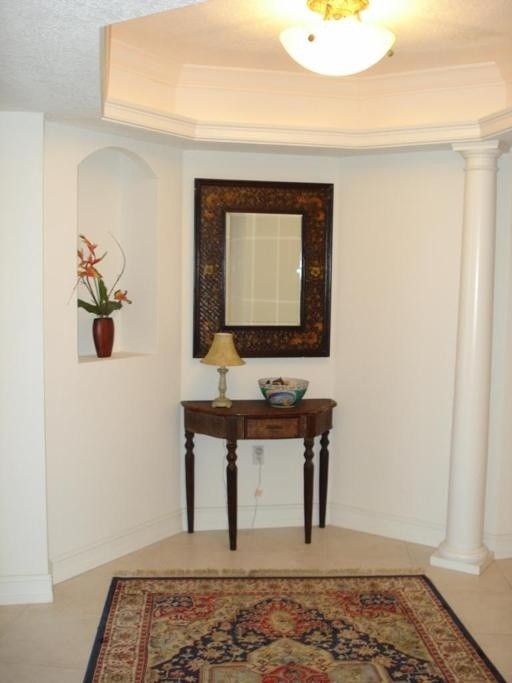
[80,568,504,682]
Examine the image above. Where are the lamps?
[274,0,399,81]
[200,331,248,410]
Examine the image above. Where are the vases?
[93,317,115,358]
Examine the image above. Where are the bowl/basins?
[259,376,309,410]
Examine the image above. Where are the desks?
[179,396,338,551]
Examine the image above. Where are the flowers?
[66,229,134,317]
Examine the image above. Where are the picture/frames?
[190,174,334,359]
